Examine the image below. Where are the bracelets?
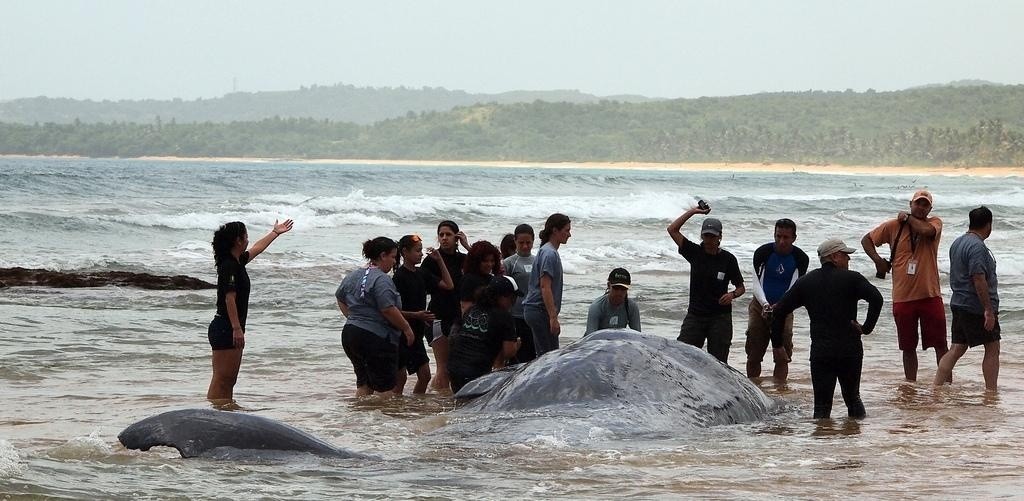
[732,289,739,298]
[903,211,910,224]
[272,230,280,237]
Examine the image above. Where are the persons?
[337,212,572,399]
[861,189,953,385]
[205,217,297,400]
[667,203,745,367]
[773,239,884,419]
[583,267,641,334]
[744,217,808,380]
[932,205,1001,388]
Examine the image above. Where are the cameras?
[876,258,893,279]
[698,199,711,215]
[763,306,774,321]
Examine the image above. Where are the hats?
[912,190,932,205]
[493,274,525,297]
[817,238,856,257]
[609,268,630,288]
[701,218,722,236]
